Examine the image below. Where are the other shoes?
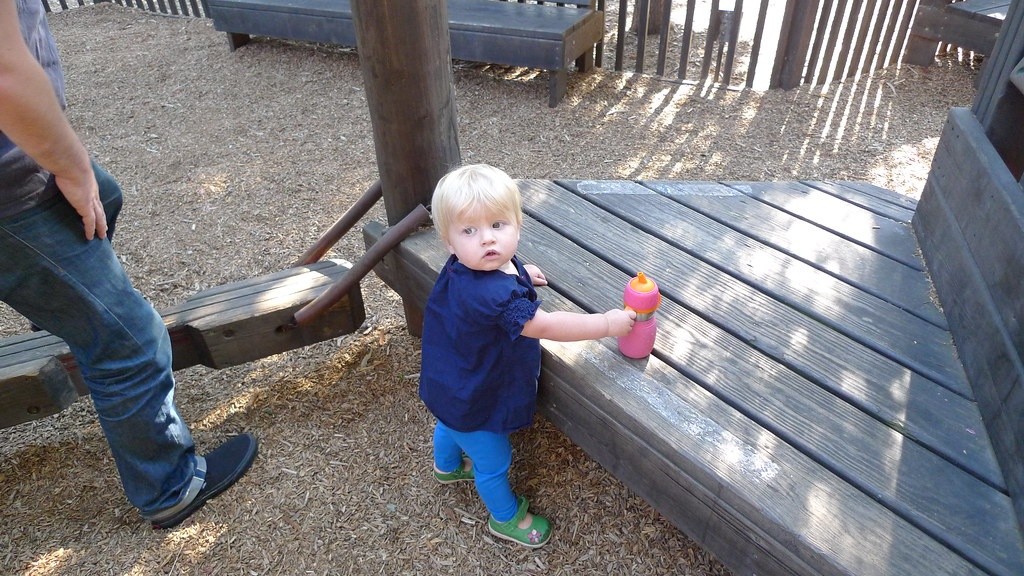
[488,497,553,548]
[433,456,475,484]
[150,434,257,529]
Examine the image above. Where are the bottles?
[616,271,662,359]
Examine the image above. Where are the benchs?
[206,0,606,108]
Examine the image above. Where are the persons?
[419,163,636,548]
[0,0,256,530]
